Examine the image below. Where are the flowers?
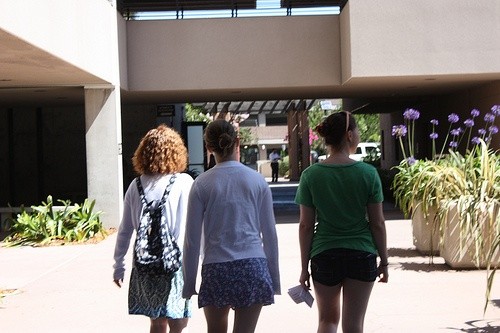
[389,105,500,314]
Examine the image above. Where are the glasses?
[339,110,352,133]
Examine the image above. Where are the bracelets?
[380,261,389,266]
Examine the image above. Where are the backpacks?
[133,173,181,275]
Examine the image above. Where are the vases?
[412,198,441,256]
[439,199,500,269]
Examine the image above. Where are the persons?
[182,119,282,333]
[269,148,284,182]
[112,125,204,333]
[294,111,389,333]
